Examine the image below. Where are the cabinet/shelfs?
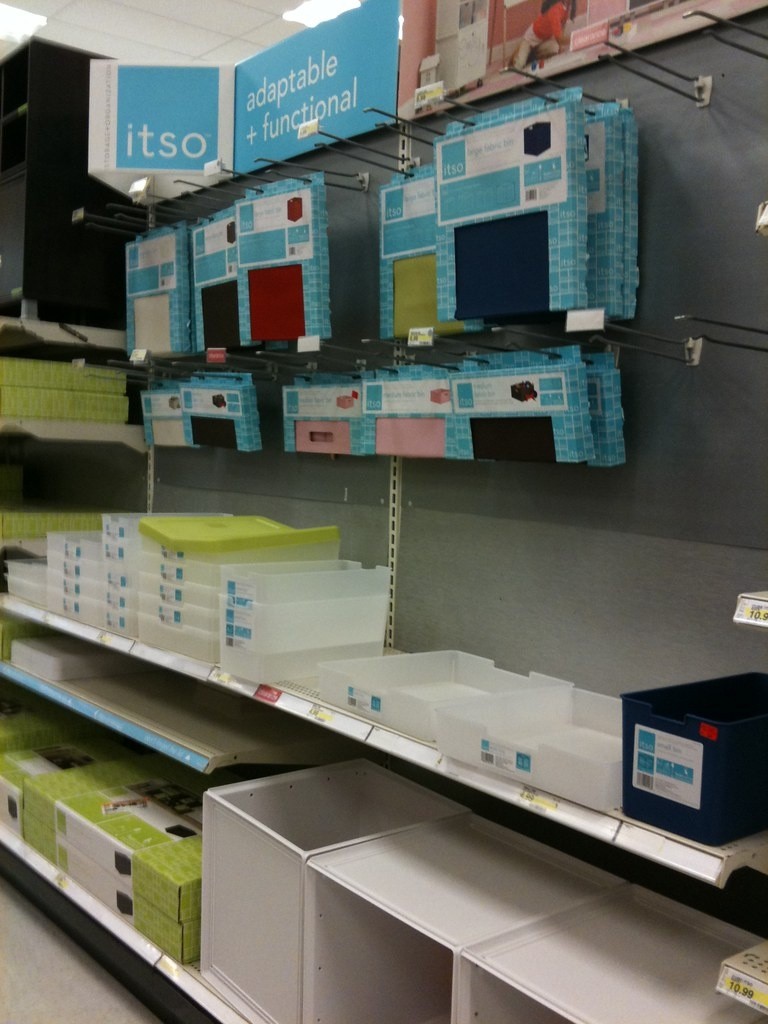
[0,593,378,968]
[155,647,767,1022]
[0,315,150,558]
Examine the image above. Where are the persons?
[513,0,575,71]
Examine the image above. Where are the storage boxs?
[1,352,767,1024]
